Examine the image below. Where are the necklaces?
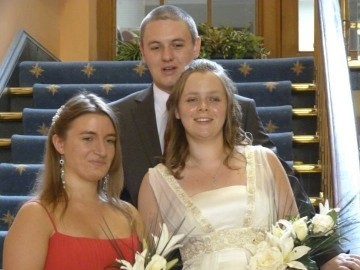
[186,148,227,185]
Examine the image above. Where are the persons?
[2,91,147,270]
[136,55,308,270]
[99,2,360,270]
